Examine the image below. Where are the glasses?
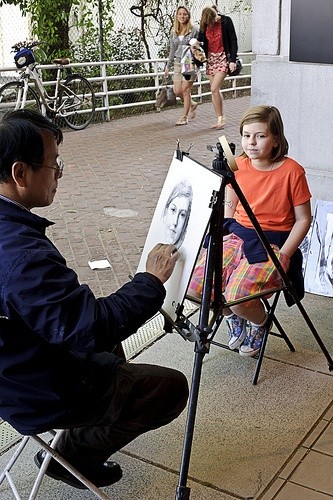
[15,155,64,171]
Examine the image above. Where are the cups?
[189,38,201,50]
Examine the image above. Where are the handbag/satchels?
[227,55,242,76]
[14,49,35,69]
[155,77,177,109]
[180,45,195,75]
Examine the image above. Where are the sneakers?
[225,313,247,350]
[239,311,273,356]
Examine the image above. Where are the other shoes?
[210,123,217,128]
[190,101,197,119]
[176,115,188,125]
[216,122,225,130]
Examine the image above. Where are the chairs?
[0,424,116,500]
[206,284,295,385]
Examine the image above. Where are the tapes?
[218,136,238,172]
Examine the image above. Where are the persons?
[191,7,238,131]
[163,6,200,126]
[0,107,190,492]
[186,104,312,359]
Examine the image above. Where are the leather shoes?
[34,440,123,489]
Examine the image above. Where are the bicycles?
[0,41,96,131]
